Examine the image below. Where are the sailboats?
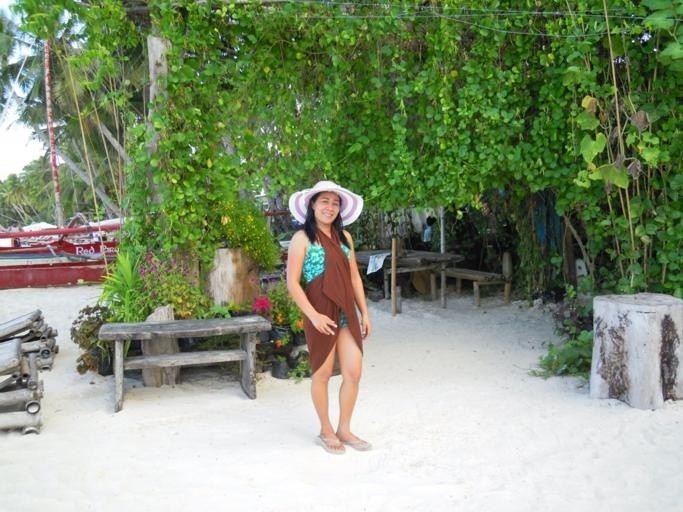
[0,22,136,291]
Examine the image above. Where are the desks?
[355,247,463,303]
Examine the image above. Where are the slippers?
[317,433,346,454]
[338,432,372,451]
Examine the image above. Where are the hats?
[288,180,364,227]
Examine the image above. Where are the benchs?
[97,313,273,413]
[434,267,505,307]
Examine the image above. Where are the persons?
[285,180,374,455]
[422,215,436,251]
